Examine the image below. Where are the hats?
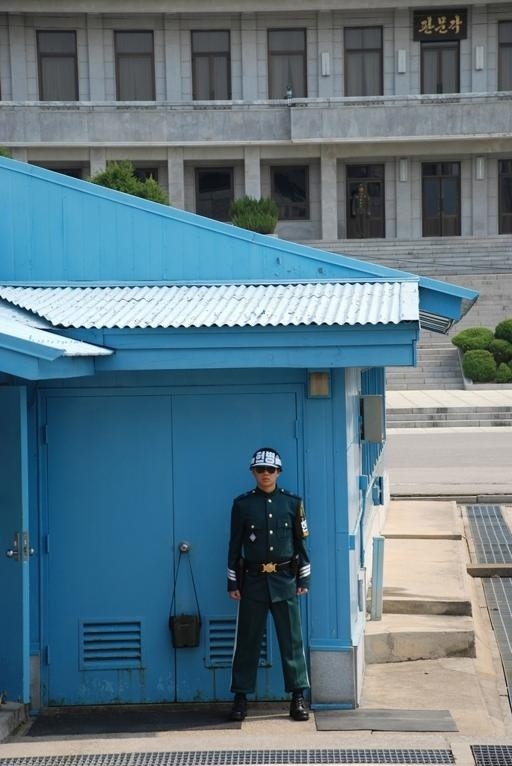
[249,447,282,471]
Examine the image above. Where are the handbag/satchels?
[168,614,202,648]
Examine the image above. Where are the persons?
[350,182,372,237]
[283,84,297,106]
[228,446,313,720]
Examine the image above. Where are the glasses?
[253,467,274,473]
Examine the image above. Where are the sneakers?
[289,696,309,721]
[230,697,247,720]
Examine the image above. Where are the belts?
[244,560,293,574]
